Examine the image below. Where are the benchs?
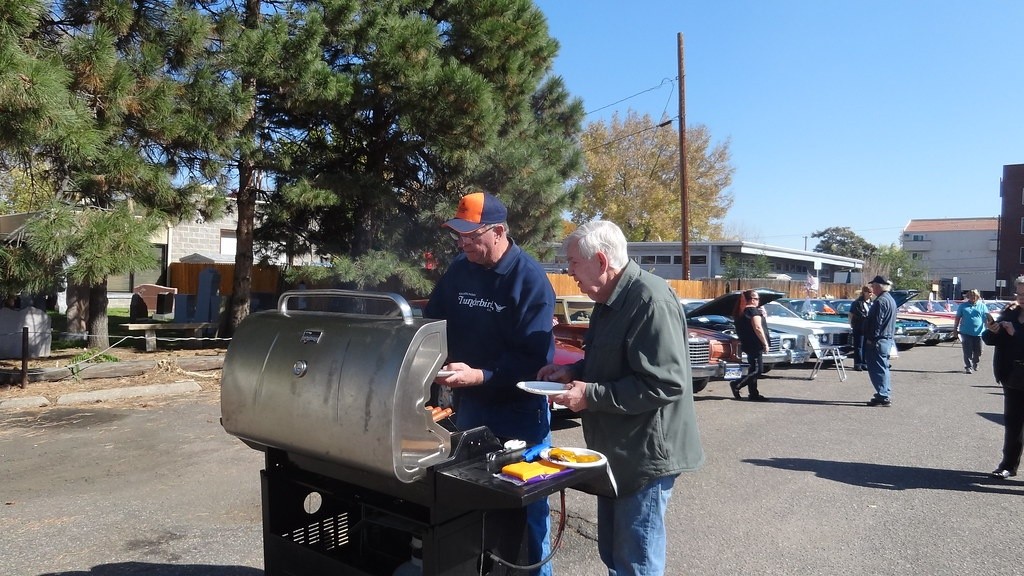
[118,321,219,352]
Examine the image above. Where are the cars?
[550,287,1023,395]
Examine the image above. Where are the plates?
[436,371,456,377]
[540,447,607,468]
[516,381,570,395]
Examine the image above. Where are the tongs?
[486,440,526,462]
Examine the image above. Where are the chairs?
[952,324,962,347]
[808,334,847,383]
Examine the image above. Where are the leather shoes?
[992,468,1011,478]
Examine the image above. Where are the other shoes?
[868,399,892,407]
[975,367,979,372]
[749,394,769,401]
[730,381,742,400]
[853,367,867,372]
[965,367,974,374]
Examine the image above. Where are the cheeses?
[501,461,545,481]
[532,459,566,474]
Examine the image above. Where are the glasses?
[753,297,760,300]
[1013,292,1024,297]
[449,226,495,244]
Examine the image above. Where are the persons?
[953,289,989,374]
[982,275,1024,478]
[421,193,556,576]
[862,274,897,407]
[537,221,705,576]
[730,290,771,400]
[848,286,873,371]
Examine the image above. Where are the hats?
[868,275,891,285]
[441,192,507,233]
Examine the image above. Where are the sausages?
[424,405,453,422]
